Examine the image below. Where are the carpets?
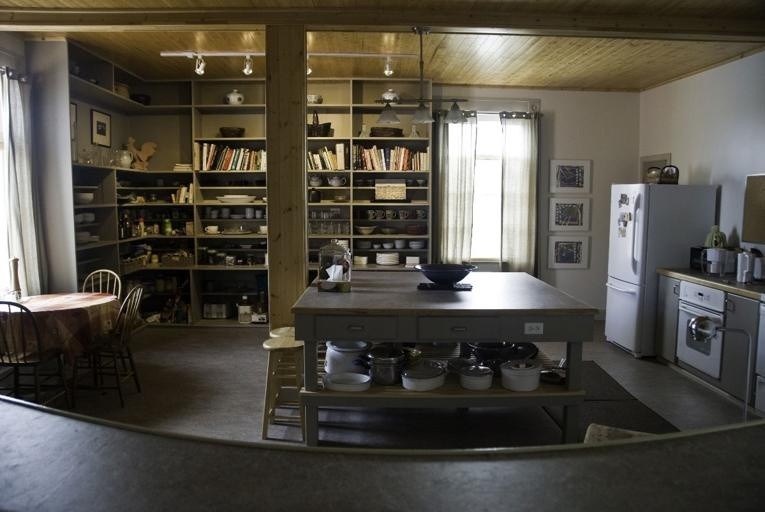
[279,355,684,453]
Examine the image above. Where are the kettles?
[736,251,755,286]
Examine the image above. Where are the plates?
[353,255,369,265]
[240,244,257,249]
[75,212,96,223]
[258,231,267,235]
[369,125,405,137]
[394,239,406,249]
[376,252,401,265]
[538,366,567,383]
[208,232,221,235]
[215,196,257,203]
[230,215,246,219]
[409,240,425,249]
[382,243,395,249]
[75,191,94,205]
[75,230,99,244]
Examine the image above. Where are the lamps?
[373,26,472,125]
[161,51,420,77]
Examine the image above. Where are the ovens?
[675,281,727,380]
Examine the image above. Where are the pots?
[308,188,320,202]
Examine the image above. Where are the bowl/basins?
[405,223,427,234]
[307,94,322,104]
[355,224,377,235]
[415,263,479,284]
[374,244,381,248]
[220,126,246,137]
[113,83,151,106]
[381,226,398,234]
[336,196,345,202]
[323,341,542,392]
[357,240,372,248]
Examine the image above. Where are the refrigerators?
[602,183,721,361]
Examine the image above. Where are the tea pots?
[327,174,346,186]
[118,148,134,169]
[308,174,322,186]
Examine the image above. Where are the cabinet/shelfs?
[194,79,352,328]
[113,62,197,324]
[722,291,760,411]
[23,35,120,292]
[654,274,680,368]
[352,78,432,269]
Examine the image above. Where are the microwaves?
[689,243,736,274]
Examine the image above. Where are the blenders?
[699,246,727,276]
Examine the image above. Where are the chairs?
[82,270,129,377]
[72,285,145,408]
[1,301,76,412]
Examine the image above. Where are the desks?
[0,291,119,398]
[291,270,598,447]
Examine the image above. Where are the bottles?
[308,222,350,236]
[238,293,254,323]
[309,209,336,219]
[408,124,421,137]
[358,124,370,137]
[154,273,178,292]
[705,225,727,246]
[118,211,194,265]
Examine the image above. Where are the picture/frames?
[550,158,593,194]
[549,197,591,232]
[547,236,590,269]
[640,153,672,182]
[90,109,112,148]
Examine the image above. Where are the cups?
[245,208,262,218]
[366,209,409,219]
[204,224,219,232]
[416,209,426,219]
[204,207,230,218]
[259,225,268,232]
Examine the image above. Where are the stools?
[270,325,300,337]
[263,336,307,443]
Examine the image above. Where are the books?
[172,143,430,172]
